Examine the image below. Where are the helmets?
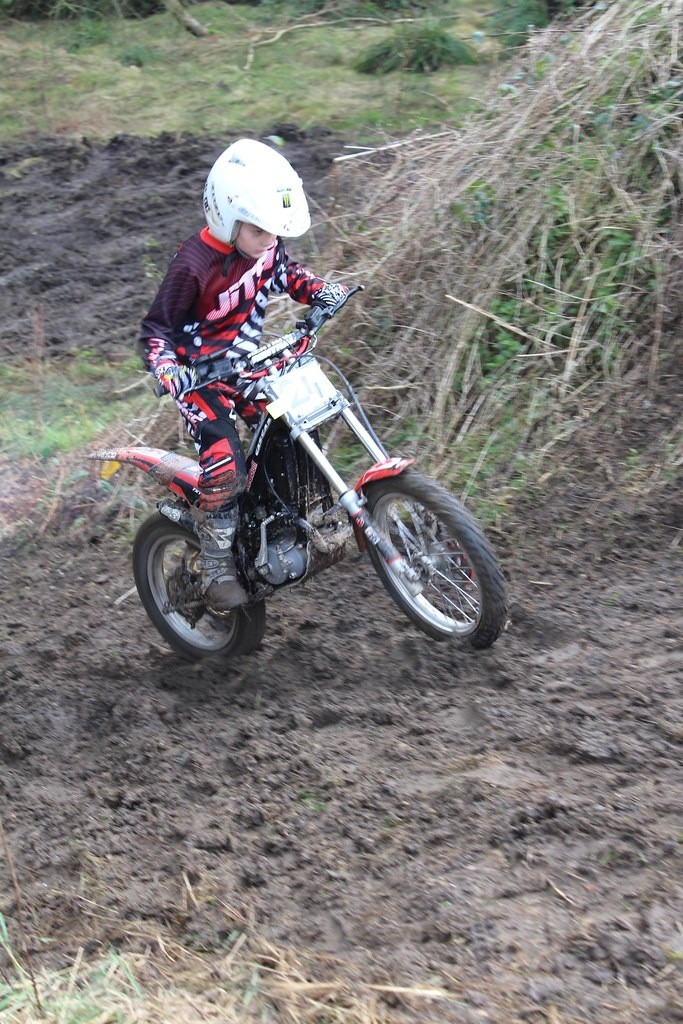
[202,139,311,244]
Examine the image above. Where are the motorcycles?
[80,285,510,664]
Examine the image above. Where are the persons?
[136,137,352,619]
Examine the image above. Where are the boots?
[193,499,247,608]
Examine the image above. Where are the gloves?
[153,358,200,401]
[314,281,350,310]
